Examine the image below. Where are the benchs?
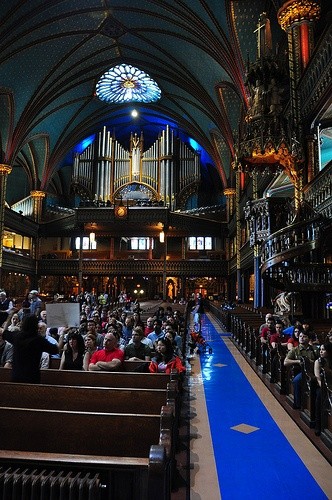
[210,301,332,450]
[0,299,187,500]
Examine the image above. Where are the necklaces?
[73,357,76,359]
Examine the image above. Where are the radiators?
[0,466,107,500]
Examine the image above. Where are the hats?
[29,290,39,294]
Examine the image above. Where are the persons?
[220,302,332,437]
[0,290,210,383]
[168,283,173,299]
[128,250,160,260]
[16,249,58,259]
